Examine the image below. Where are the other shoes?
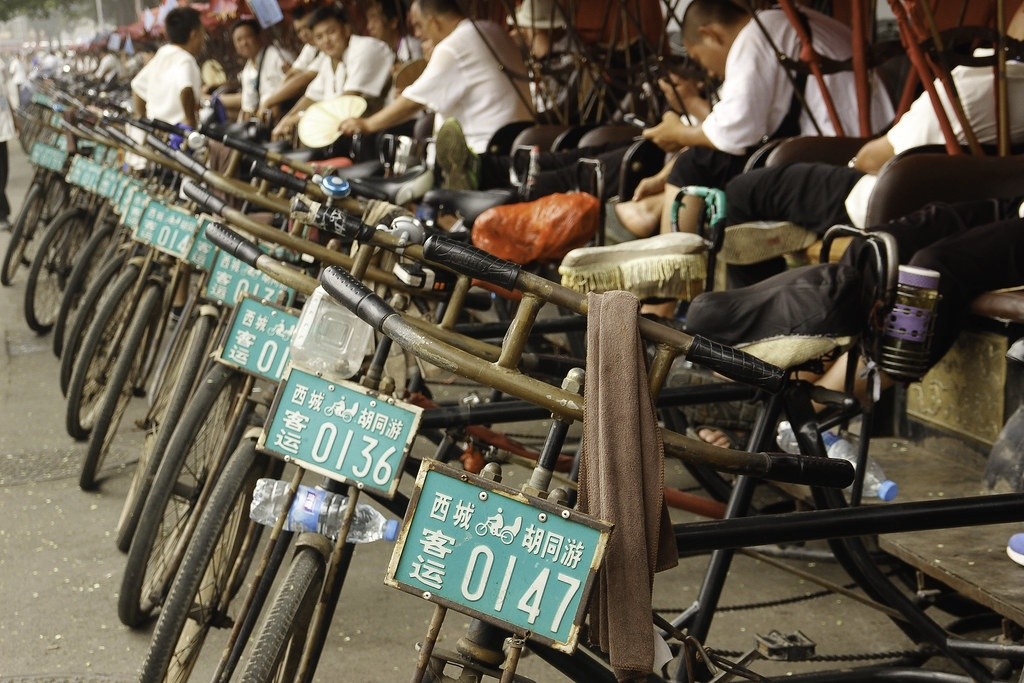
[1006,533,1024,565]
[168,305,204,332]
[598,196,637,245]
[0,219,12,231]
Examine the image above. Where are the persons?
[0,0,1024,566]
[338,0,536,185]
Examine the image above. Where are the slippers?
[667,368,733,385]
[684,425,753,451]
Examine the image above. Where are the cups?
[883,265,941,374]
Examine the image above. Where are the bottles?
[250,478,398,543]
[778,420,898,500]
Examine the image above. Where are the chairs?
[493,126,1024,628]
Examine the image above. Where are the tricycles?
[1,44,1021,681]
